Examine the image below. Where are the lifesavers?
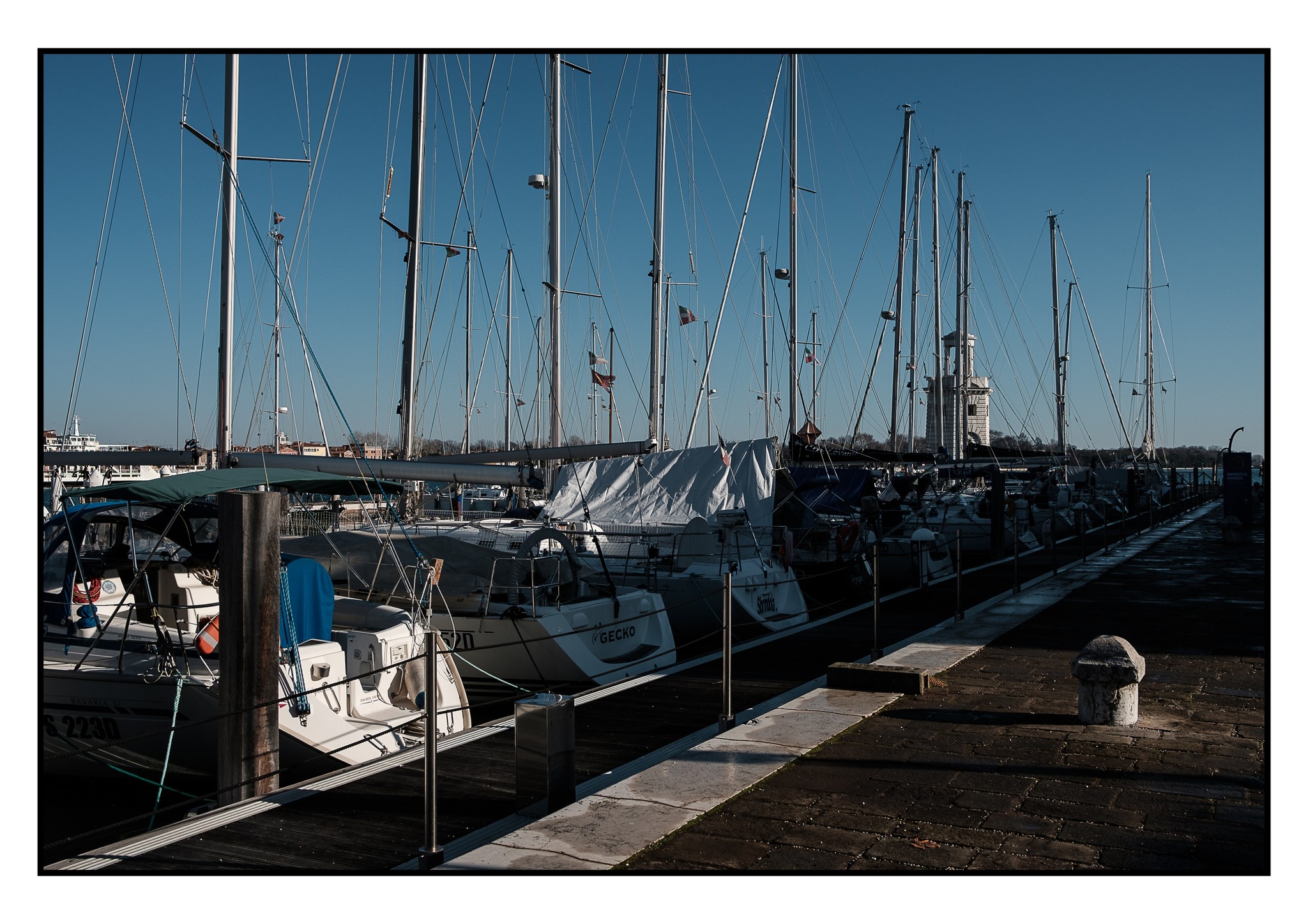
[837,521,860,550]
[781,532,793,572]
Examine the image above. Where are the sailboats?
[42,50,1223,818]
